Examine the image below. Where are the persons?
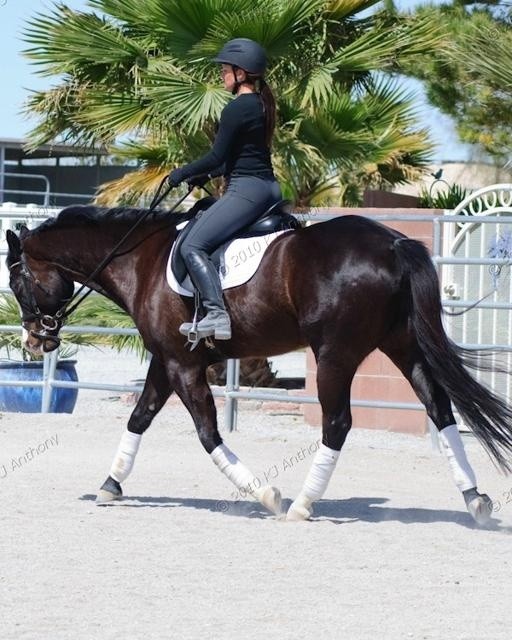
[165,36,286,343]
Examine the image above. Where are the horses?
[4,204,512,527]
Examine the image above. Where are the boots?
[177,249,234,341]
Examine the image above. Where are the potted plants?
[1,290,110,413]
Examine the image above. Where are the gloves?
[168,164,197,189]
[185,173,212,189]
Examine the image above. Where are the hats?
[210,37,268,76]
